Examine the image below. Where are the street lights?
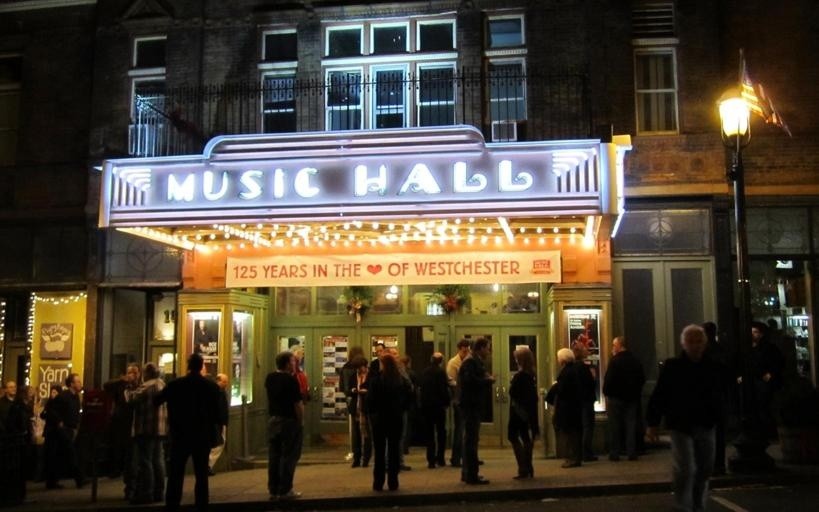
[716,84,752,330]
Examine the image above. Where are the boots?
[353,443,371,467]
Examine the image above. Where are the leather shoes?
[513,475,528,480]
[477,475,490,484]
[561,455,598,468]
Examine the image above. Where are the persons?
[340,339,494,489]
[550,348,585,468]
[574,343,602,462]
[290,345,311,431]
[644,323,726,512]
[702,321,731,473]
[155,353,227,509]
[0,363,167,509]
[507,349,538,479]
[746,322,783,380]
[265,352,303,498]
[603,338,646,460]
[207,373,229,476]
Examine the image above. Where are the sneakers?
[400,464,411,470]
[428,459,483,467]
[270,489,303,499]
